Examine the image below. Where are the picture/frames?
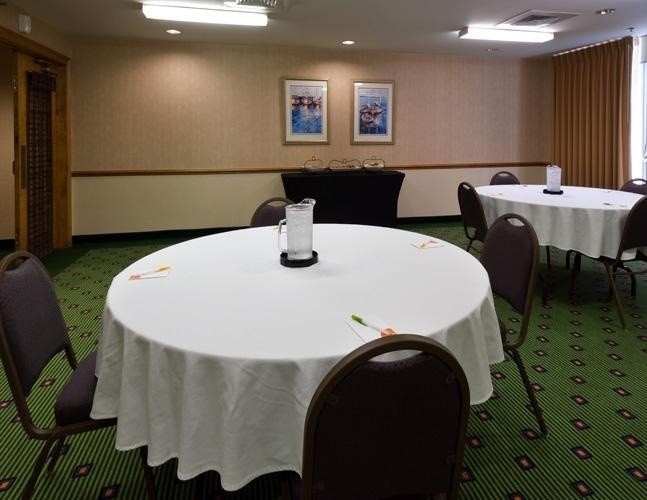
[351,80,395,144]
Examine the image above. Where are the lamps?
[281,75,330,145]
[137,0,271,27]
[459,27,554,44]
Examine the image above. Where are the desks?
[281,170,405,228]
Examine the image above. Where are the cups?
[547,166,562,192]
[278,197,316,260]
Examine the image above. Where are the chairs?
[480,213,547,436]
[281,335,470,500]
[0,251,157,500]
[620,178,647,195]
[250,198,294,227]
[91,224,505,498]
[570,195,647,329]
[457,181,548,304]
[490,171,520,184]
